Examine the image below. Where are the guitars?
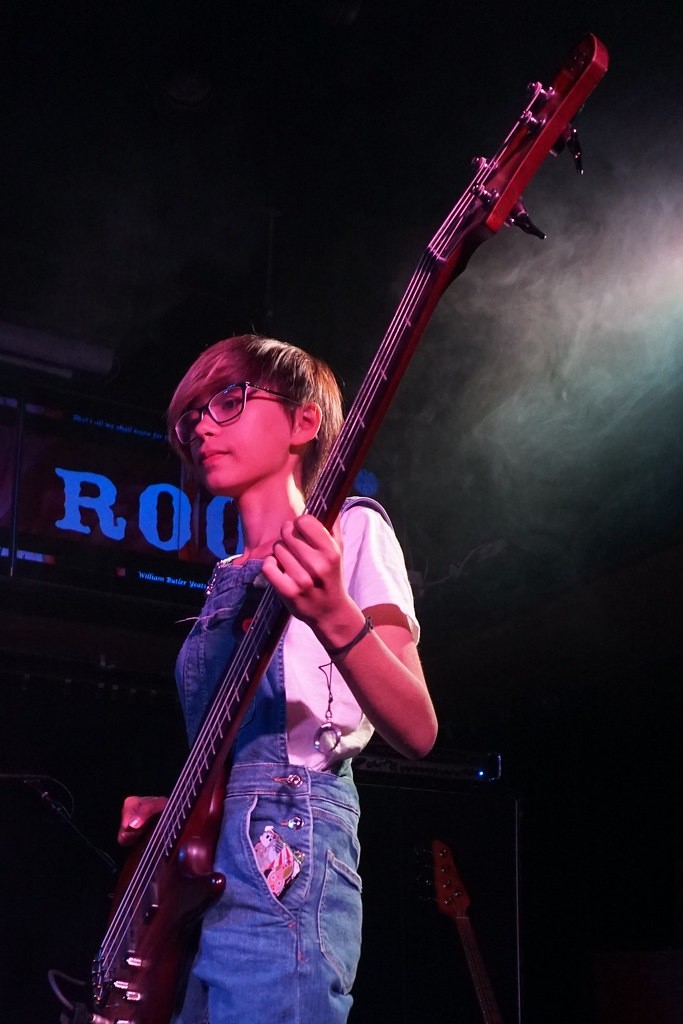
[57,27,611,1024]
[412,836,505,1023]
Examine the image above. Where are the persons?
[119,334,439,1024]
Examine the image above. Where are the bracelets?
[325,617,373,656]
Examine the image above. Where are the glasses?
[173,379,301,444]
